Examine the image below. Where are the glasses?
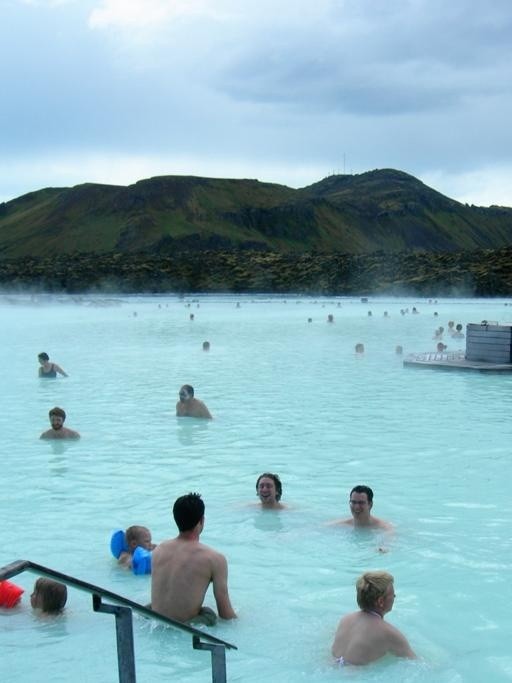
[349,500,367,504]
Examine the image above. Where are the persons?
[176,384,214,418]
[432,330,439,338]
[148,492,237,625]
[327,484,397,556]
[439,325,444,334]
[1,580,25,608]
[119,524,157,567]
[248,473,287,510]
[437,342,447,352]
[331,570,416,668]
[203,341,211,350]
[395,345,403,352]
[27,575,70,617]
[41,406,81,439]
[447,320,455,332]
[355,342,363,353]
[451,323,465,337]
[38,352,68,376]
